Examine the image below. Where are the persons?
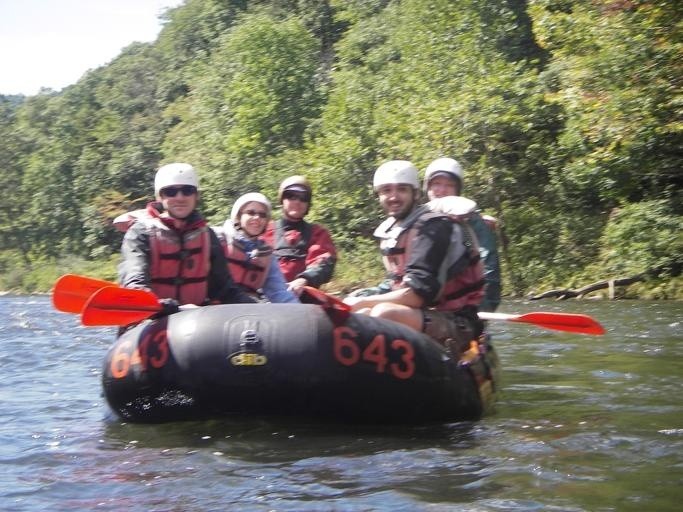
[255,173,336,305]
[209,190,305,305]
[342,158,485,355]
[421,156,502,314]
[114,161,257,339]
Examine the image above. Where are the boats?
[98,302,498,427]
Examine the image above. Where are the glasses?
[159,184,198,198]
[240,209,268,219]
[280,188,312,203]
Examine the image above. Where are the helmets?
[423,155,466,194]
[154,161,200,203]
[230,190,272,227]
[371,158,421,191]
[277,173,313,207]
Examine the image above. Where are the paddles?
[478,312,608,335]
[53,275,124,312]
[81,288,199,326]
[300,285,352,312]
